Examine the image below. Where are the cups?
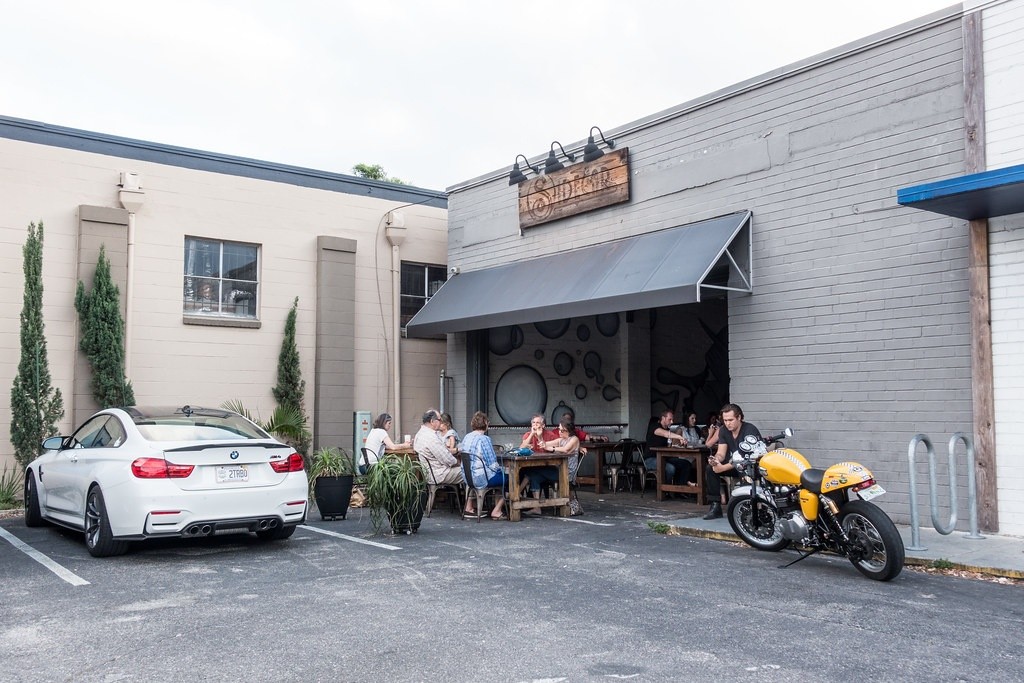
[404,434,411,443]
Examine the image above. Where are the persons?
[643,410,733,505]
[412,410,610,521]
[359,413,412,476]
[702,404,761,520]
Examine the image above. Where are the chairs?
[413,452,463,516]
[538,449,585,517]
[604,437,676,499]
[458,451,510,523]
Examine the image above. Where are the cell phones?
[707,456,716,460]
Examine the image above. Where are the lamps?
[545,141,575,174]
[584,126,616,163]
[509,154,540,185]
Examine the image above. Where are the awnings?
[405,209,753,338]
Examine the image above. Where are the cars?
[23,406,308,559]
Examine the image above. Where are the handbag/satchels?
[509,447,533,456]
[351,485,367,507]
[570,498,584,516]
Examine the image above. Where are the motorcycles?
[725,427,906,581]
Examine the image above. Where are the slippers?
[522,511,538,518]
[492,513,508,520]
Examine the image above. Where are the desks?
[385,450,421,483]
[496,452,575,522]
[575,440,646,493]
[649,446,718,505]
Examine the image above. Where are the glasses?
[558,428,567,433]
[436,418,442,422]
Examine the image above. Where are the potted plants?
[365,451,431,538]
[309,444,354,523]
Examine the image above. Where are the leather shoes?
[704,502,723,520]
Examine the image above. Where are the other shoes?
[465,509,488,518]
[674,492,688,499]
